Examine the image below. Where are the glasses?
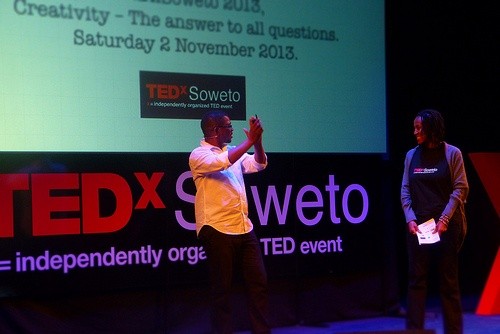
[217,125,232,128]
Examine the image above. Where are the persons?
[400,108,470,334]
[189,111,272,334]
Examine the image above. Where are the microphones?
[203,135,218,140]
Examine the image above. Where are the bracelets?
[439,214,449,228]
